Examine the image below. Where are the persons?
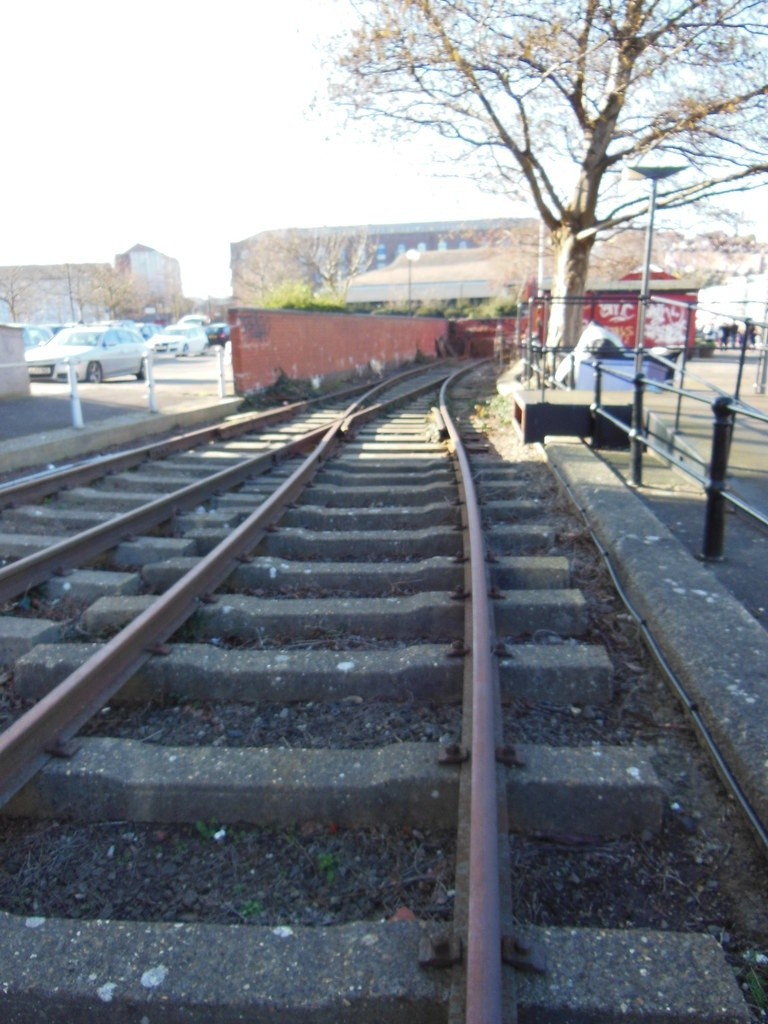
[704,318,761,350]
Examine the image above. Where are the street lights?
[627,156,691,489]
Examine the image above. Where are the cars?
[22,312,232,348]
[144,324,211,359]
[25,324,148,384]
